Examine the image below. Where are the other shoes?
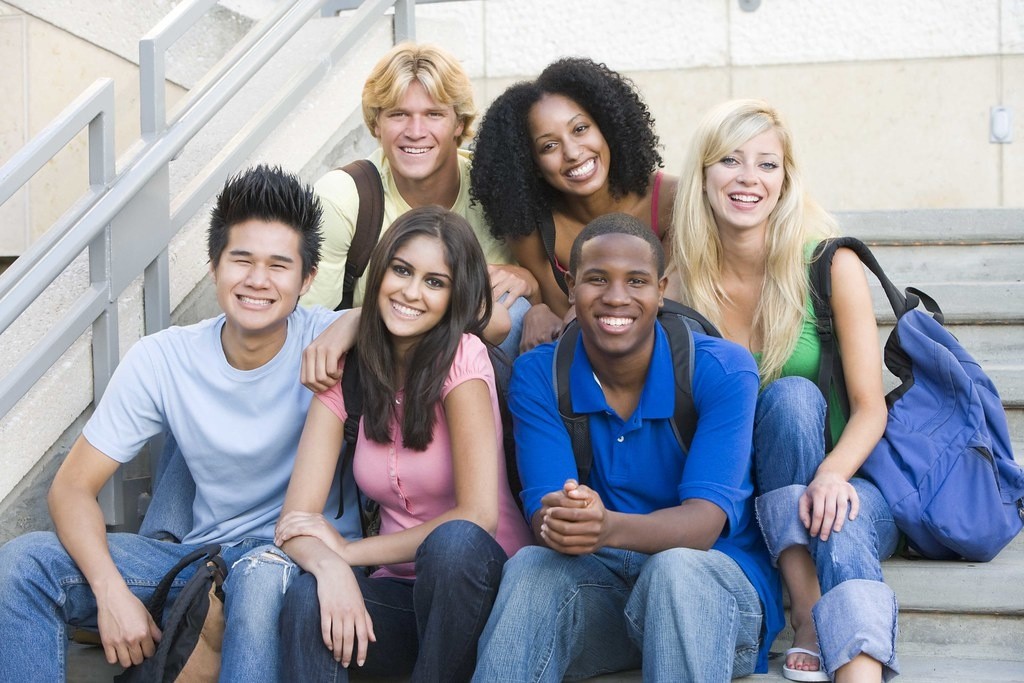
[783,643,831,683]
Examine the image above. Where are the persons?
[0,43,785,683]
[662,96,899,683]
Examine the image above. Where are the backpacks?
[116,542,231,682]
[809,233,1023,564]
[553,296,724,492]
